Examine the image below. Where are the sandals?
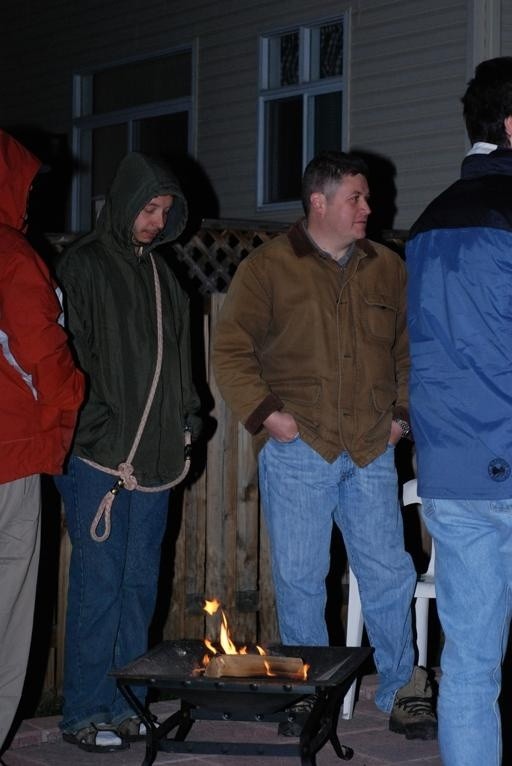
[61,721,131,753]
[116,717,162,742]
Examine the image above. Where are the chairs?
[342,479,436,722]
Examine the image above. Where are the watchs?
[393,417,409,437]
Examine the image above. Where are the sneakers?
[388,687,438,741]
[278,698,319,736]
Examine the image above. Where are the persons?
[401,51,511,765]
[0,130,90,755]
[49,145,222,753]
[200,152,436,743]
